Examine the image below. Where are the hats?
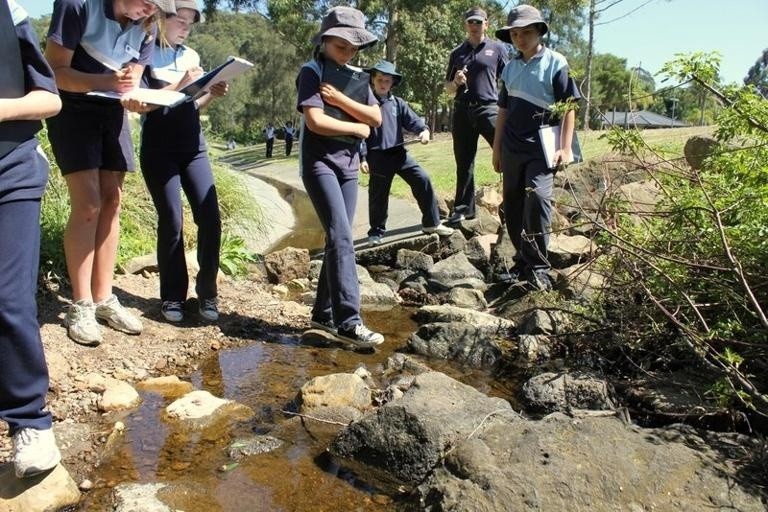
[309,4,382,50]
[149,0,179,16]
[464,6,488,21]
[364,60,403,88]
[175,0,201,24]
[495,4,548,42]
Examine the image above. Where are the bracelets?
[454,80,460,89]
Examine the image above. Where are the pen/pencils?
[103,63,121,73]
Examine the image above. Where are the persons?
[0,1,61,480]
[361,60,454,247]
[282,121,297,156]
[293,6,384,348]
[492,5,581,290]
[137,0,228,323]
[442,7,510,229]
[41,0,178,347]
[262,122,275,158]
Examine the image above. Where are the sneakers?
[529,274,552,293]
[62,299,103,347]
[421,225,454,236]
[310,314,338,335]
[496,267,522,281]
[197,296,219,321]
[368,236,382,247]
[160,300,185,323]
[448,212,475,224]
[11,424,63,479]
[338,321,384,348]
[92,294,145,334]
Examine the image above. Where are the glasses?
[467,20,487,25]
